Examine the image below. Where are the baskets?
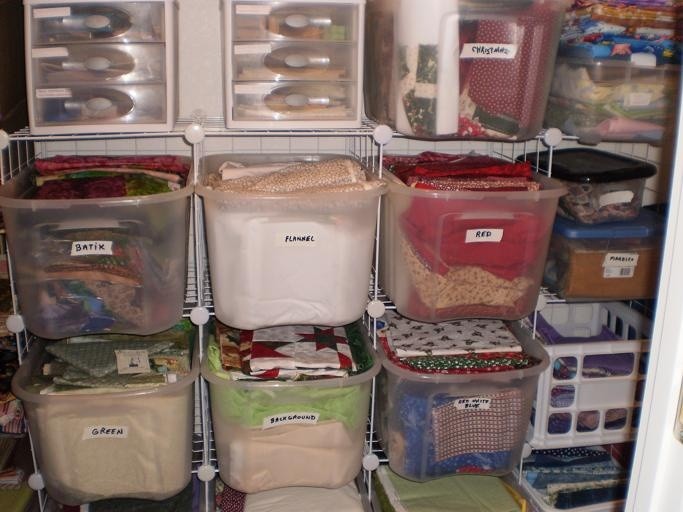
[519,302,654,449]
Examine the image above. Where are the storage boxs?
[0,0,682,512]
